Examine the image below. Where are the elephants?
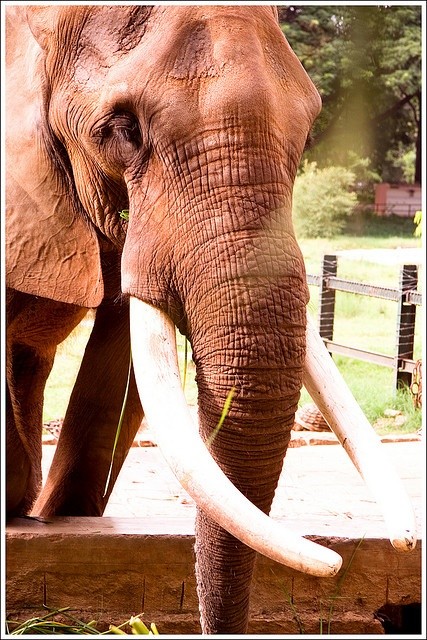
[5,5,417,635]
